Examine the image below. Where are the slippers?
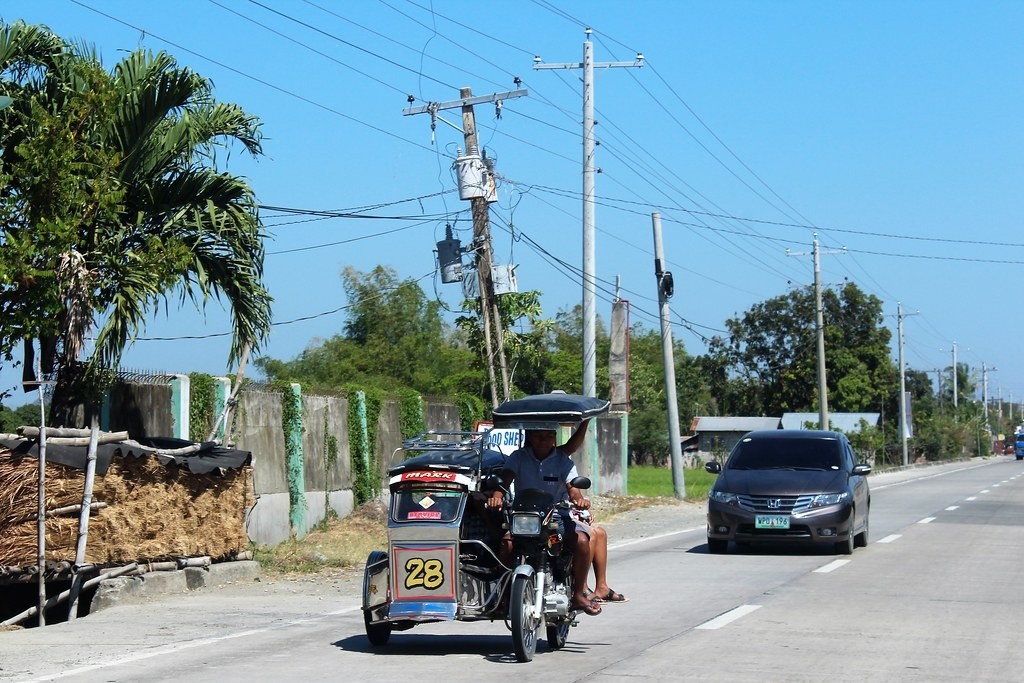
[577,601,602,616]
[583,588,608,605]
[603,588,630,603]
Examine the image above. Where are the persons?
[487,430,602,616]
[555,418,629,604]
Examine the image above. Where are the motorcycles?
[487,475,593,662]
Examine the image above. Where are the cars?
[705,429,872,555]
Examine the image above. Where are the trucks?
[1015,433,1024,460]
